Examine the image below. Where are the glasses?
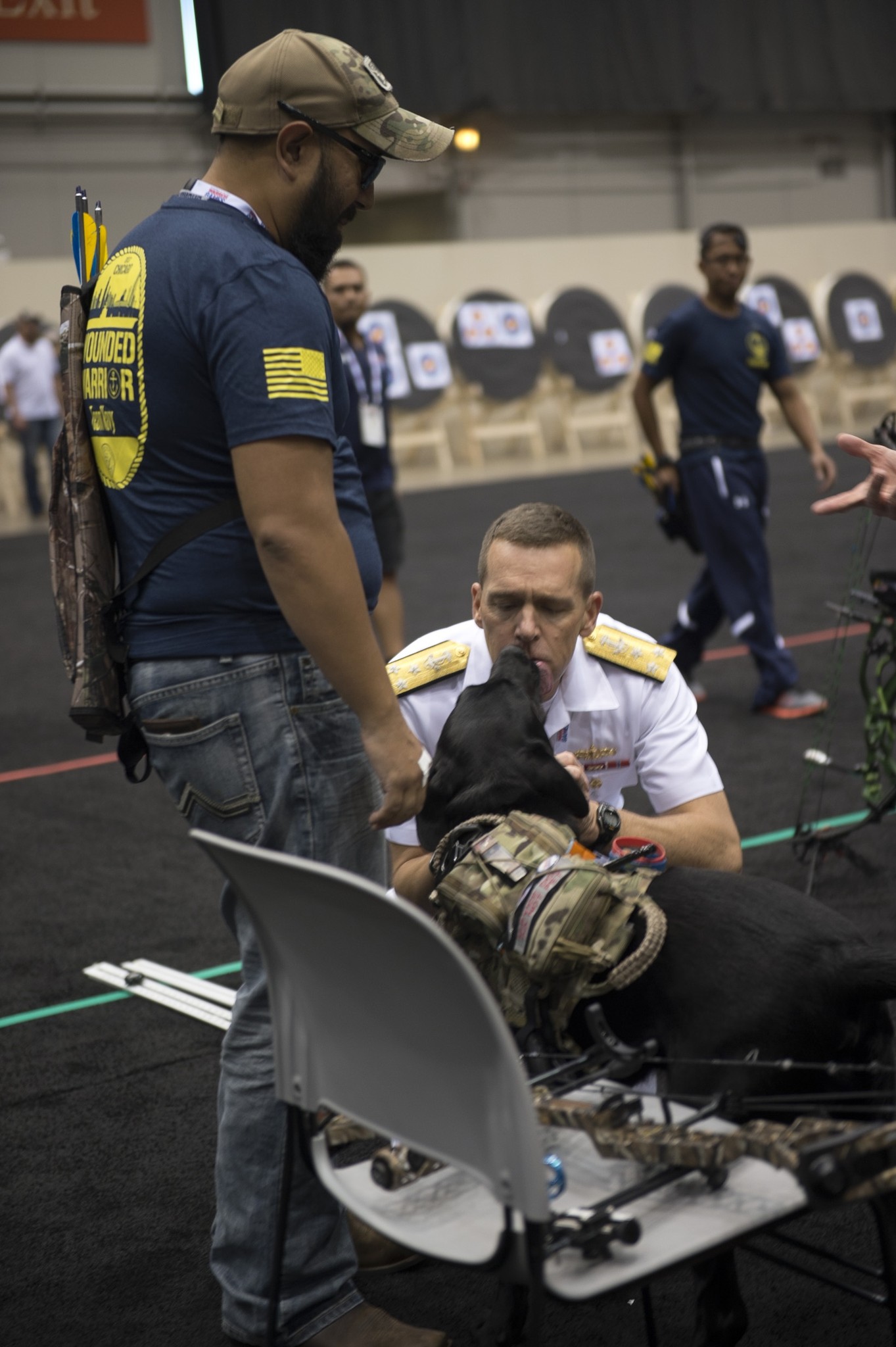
[278,100,386,189]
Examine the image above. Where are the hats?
[212,28,455,163]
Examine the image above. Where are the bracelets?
[658,456,674,468]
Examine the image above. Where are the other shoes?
[340,1214,423,1278]
[222,1300,452,1347]
[757,686,829,719]
[686,676,706,706]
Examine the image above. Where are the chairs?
[388,351,896,472]
[188,829,809,1347]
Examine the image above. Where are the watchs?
[588,797,622,854]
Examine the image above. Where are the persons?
[0,305,64,520]
[383,503,745,907]
[631,222,828,721]
[808,430,896,515]
[324,259,405,659]
[80,28,458,1347]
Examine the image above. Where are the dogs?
[407,628,896,1131]
